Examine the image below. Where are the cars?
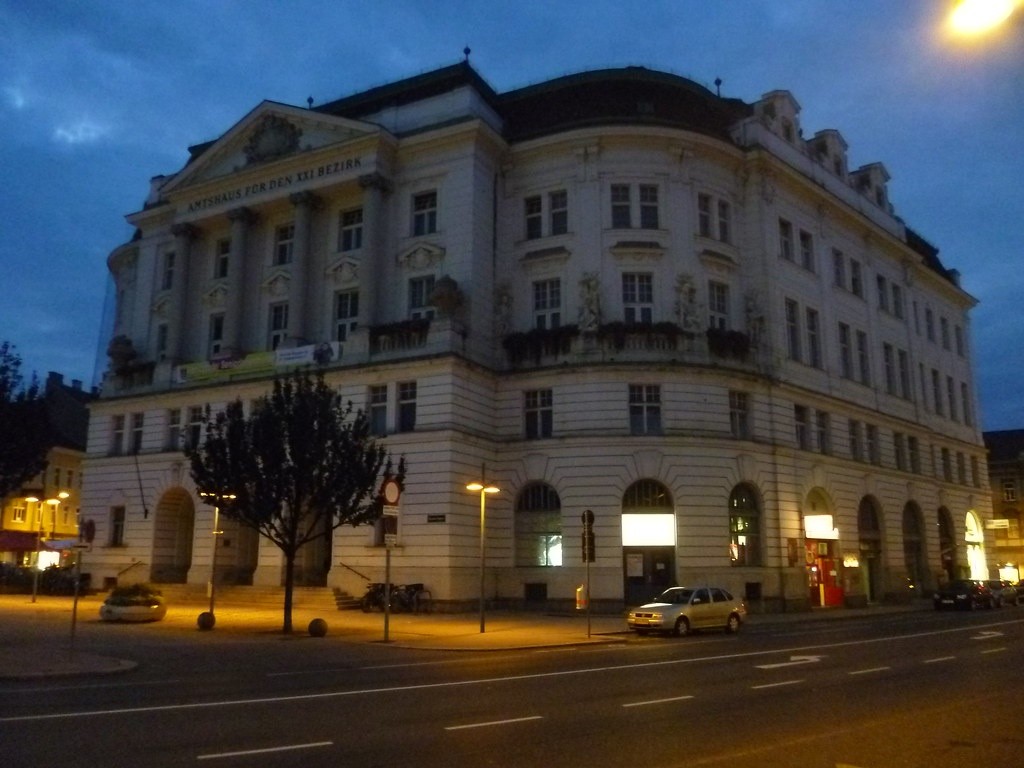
[932,578,1024,612]
[626,584,747,638]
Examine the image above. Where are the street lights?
[199,487,237,614]
[25,489,60,604]
[52,490,70,541]
[466,462,501,633]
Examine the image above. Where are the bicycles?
[360,580,434,617]
